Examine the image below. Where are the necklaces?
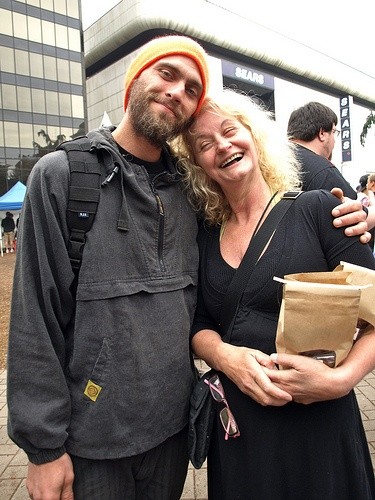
[249,191,280,244]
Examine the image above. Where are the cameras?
[298,350,336,369]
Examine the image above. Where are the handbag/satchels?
[187,381,214,470]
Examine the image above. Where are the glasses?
[331,128,340,137]
[204,375,240,440]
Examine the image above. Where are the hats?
[358,175,369,192]
[123,36,209,119]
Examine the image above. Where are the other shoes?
[10,249,14,253]
[6,250,9,253]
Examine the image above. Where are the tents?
[0,181,27,257]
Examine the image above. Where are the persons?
[7,36,372,500]
[171,87,375,500]
[1,212,21,254]
[286,102,375,256]
